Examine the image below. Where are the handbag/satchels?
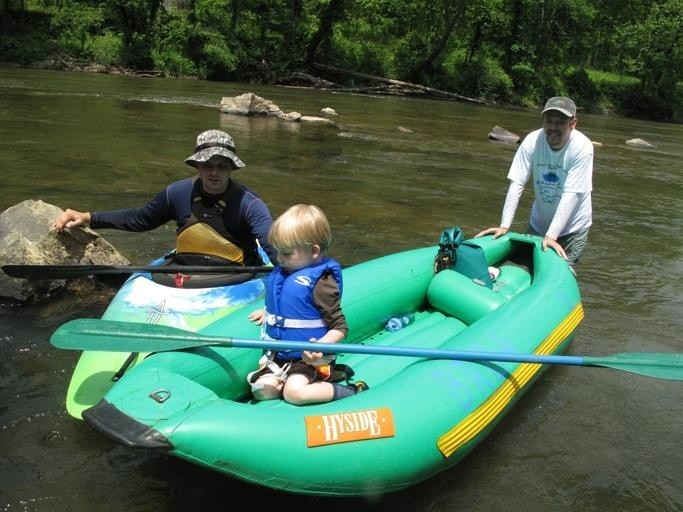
[438,226,494,291]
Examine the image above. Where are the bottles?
[487,267,502,280]
[384,311,415,331]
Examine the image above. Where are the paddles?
[0,264,271,280]
[50,318,682,380]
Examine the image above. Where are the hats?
[183,129,246,169]
[540,96,578,119]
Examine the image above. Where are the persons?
[474,97,594,268]
[56,128,282,277]
[250,204,368,406]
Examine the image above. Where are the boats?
[78,229,584,501]
[61,233,275,423]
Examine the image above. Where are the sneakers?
[350,380,369,396]
